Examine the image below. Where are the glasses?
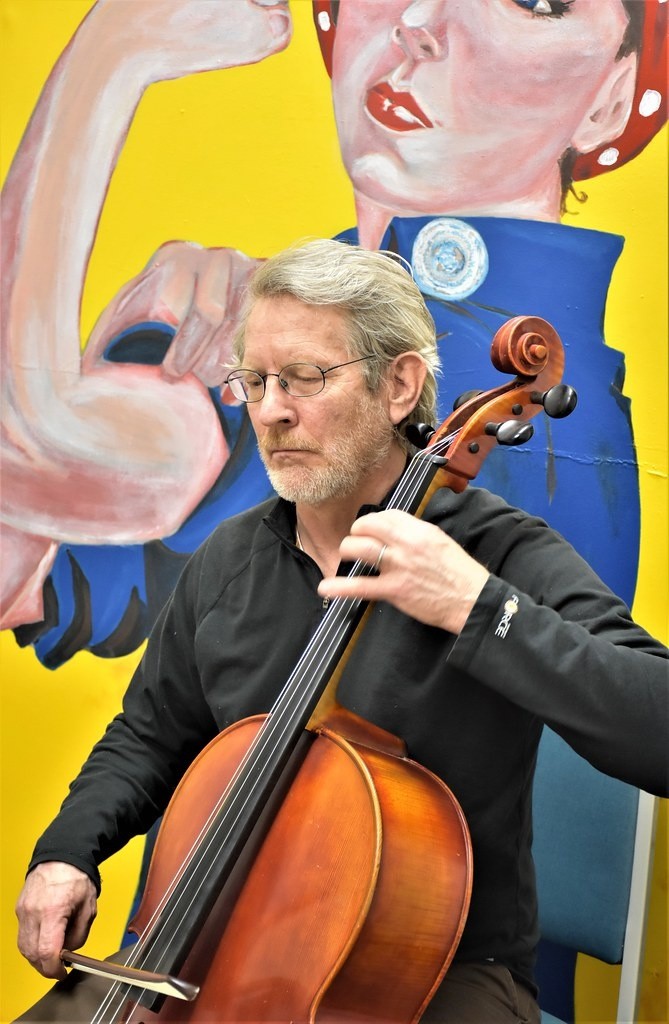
[224,356,374,403]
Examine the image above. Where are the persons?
[11,239,669,1024]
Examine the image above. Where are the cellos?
[10,315,577,1024]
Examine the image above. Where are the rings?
[376,544,387,570]
